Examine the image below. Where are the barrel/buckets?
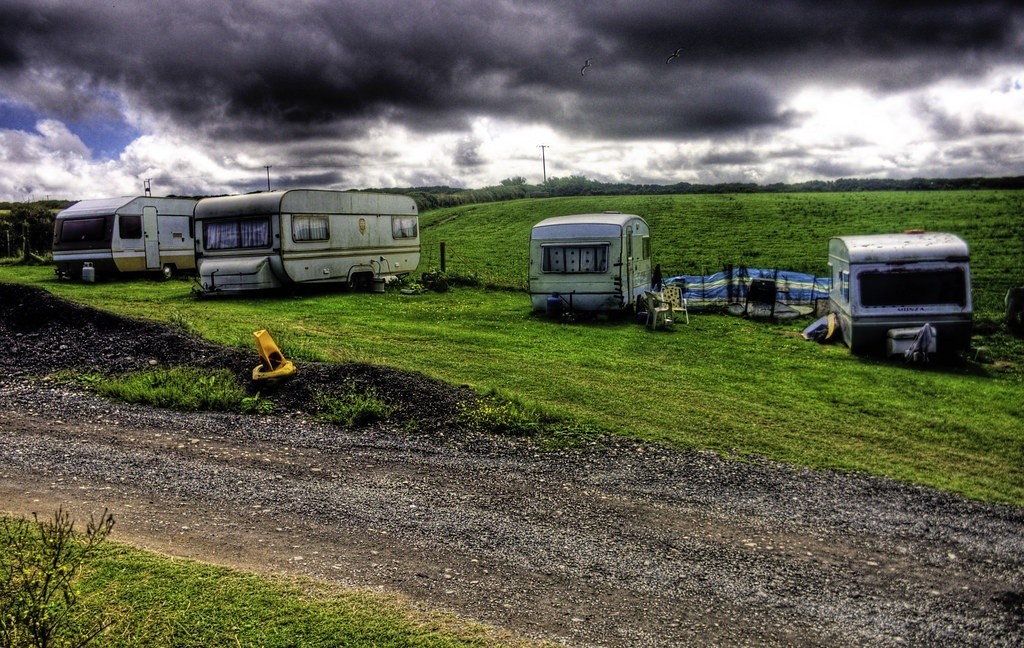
[373,277,385,293]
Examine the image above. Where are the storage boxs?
[887,327,938,353]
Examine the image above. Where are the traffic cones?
[251,329,297,377]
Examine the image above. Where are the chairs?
[644,291,674,330]
[660,287,690,326]
